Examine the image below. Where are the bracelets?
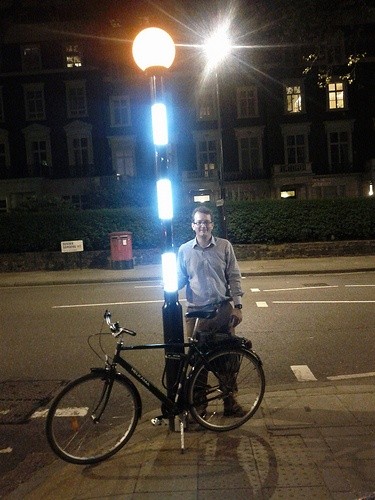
[234,305,244,310]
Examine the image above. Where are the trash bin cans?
[108,231,134,269]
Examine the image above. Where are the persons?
[170,206,250,420]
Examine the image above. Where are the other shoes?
[223,406,247,417]
[200,411,208,418]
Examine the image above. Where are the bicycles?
[45,311,266,466]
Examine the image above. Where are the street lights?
[131,28,176,413]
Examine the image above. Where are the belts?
[194,300,226,310]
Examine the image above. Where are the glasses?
[194,221,213,225]
